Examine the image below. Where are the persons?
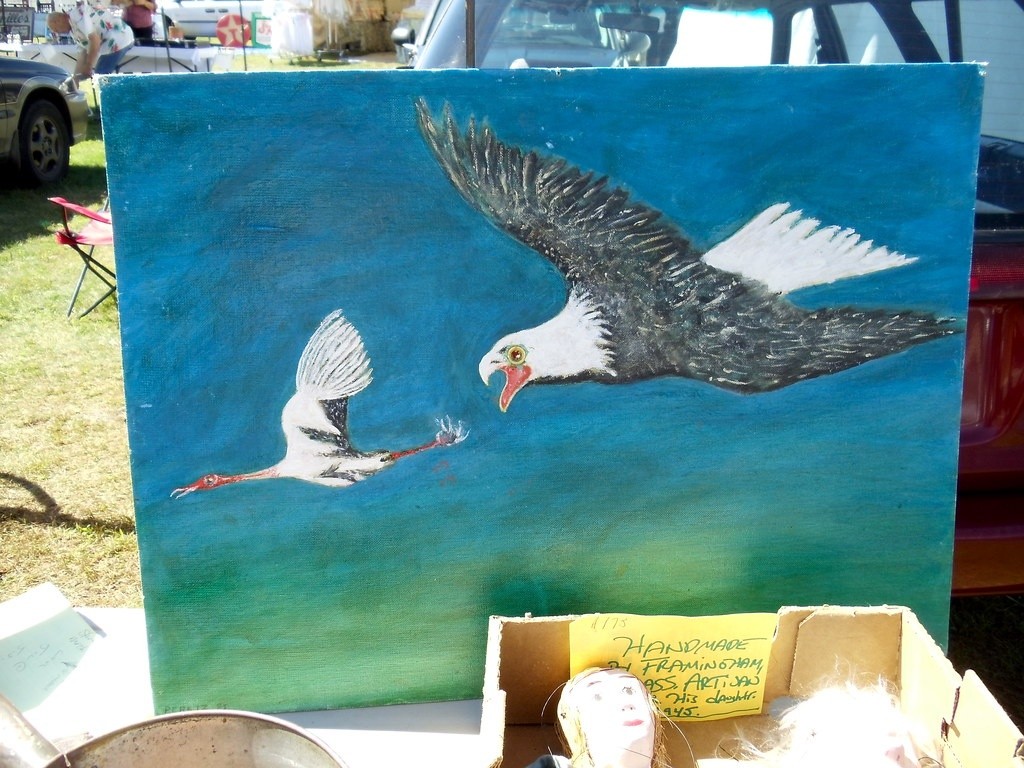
[110,0,157,74]
[47,5,135,78]
[556,666,659,768]
[792,687,919,768]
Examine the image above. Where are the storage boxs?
[478,604,1024,768]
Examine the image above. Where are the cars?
[1,57,87,182]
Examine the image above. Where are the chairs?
[47,197,118,321]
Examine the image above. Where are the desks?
[0,607,483,768]
[0,42,219,115]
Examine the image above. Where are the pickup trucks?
[392,3,1017,608]
